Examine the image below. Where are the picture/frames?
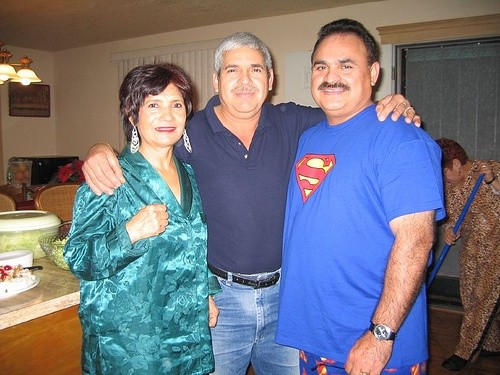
[8,81,51,118]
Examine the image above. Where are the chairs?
[33,182,80,223]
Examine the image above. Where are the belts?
[208,264,280,289]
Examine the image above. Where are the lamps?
[0,39,42,90]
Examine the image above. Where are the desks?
[0,184,36,210]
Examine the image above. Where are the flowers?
[50,157,85,184]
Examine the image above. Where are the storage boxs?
[6,155,80,189]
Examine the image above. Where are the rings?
[483,165,486,168]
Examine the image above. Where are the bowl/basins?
[39,229,71,271]
[0,249,34,267]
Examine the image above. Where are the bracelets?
[486,177,496,184]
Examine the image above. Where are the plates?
[0,274,41,300]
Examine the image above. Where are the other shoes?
[478,349,500,357]
[442,355,468,370]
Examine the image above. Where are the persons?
[64,63,224,375]
[79,30,427,375]
[274,20,500,375]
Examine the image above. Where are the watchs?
[369,320,396,341]
